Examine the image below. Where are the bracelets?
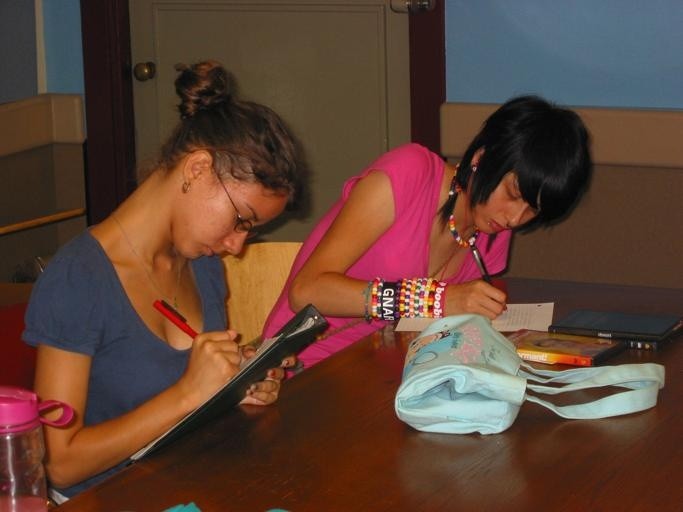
[364,275,449,327]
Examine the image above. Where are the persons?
[20,54,312,507]
[258,95,596,384]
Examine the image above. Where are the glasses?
[211,164,259,239]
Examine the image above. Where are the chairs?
[220,242,305,369]
[0,282,41,413]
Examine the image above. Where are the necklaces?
[448,161,480,250]
[111,213,182,309]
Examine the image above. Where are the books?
[505,329,625,368]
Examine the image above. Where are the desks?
[55,278,683,512]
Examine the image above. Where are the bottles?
[0,386,75,512]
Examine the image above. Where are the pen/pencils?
[470,242,491,285]
[153,300,198,338]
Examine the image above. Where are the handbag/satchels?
[394,313,666,435]
